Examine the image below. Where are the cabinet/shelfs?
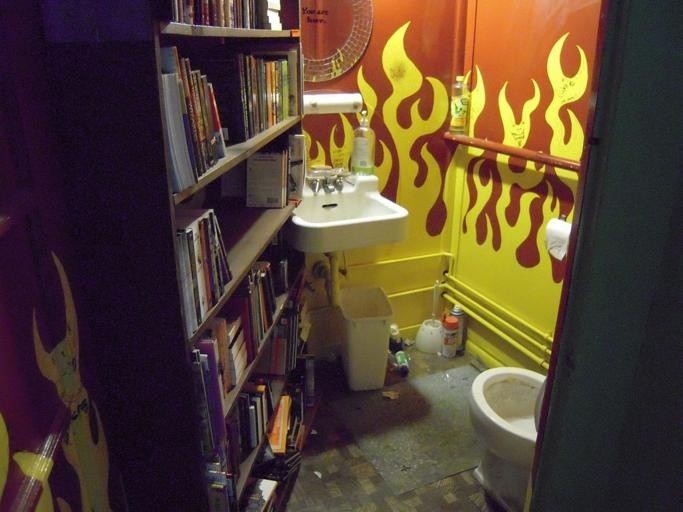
[83,2,316,512]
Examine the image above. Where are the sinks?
[291,175,409,253]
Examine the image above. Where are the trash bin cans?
[340,285,394,393]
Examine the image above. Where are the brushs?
[428,279,440,329]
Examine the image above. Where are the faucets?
[323,175,337,192]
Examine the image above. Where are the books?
[161,43,299,191]
[245,134,304,208]
[194,225,289,401]
[205,304,305,512]
[158,1,283,30]
[176,208,231,339]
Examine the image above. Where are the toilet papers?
[544,218,572,262]
[303,91,364,115]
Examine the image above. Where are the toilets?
[469,367,549,511]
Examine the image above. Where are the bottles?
[441,316,459,358]
[388,335,409,377]
[450,304,464,353]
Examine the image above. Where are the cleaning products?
[352,110,375,174]
